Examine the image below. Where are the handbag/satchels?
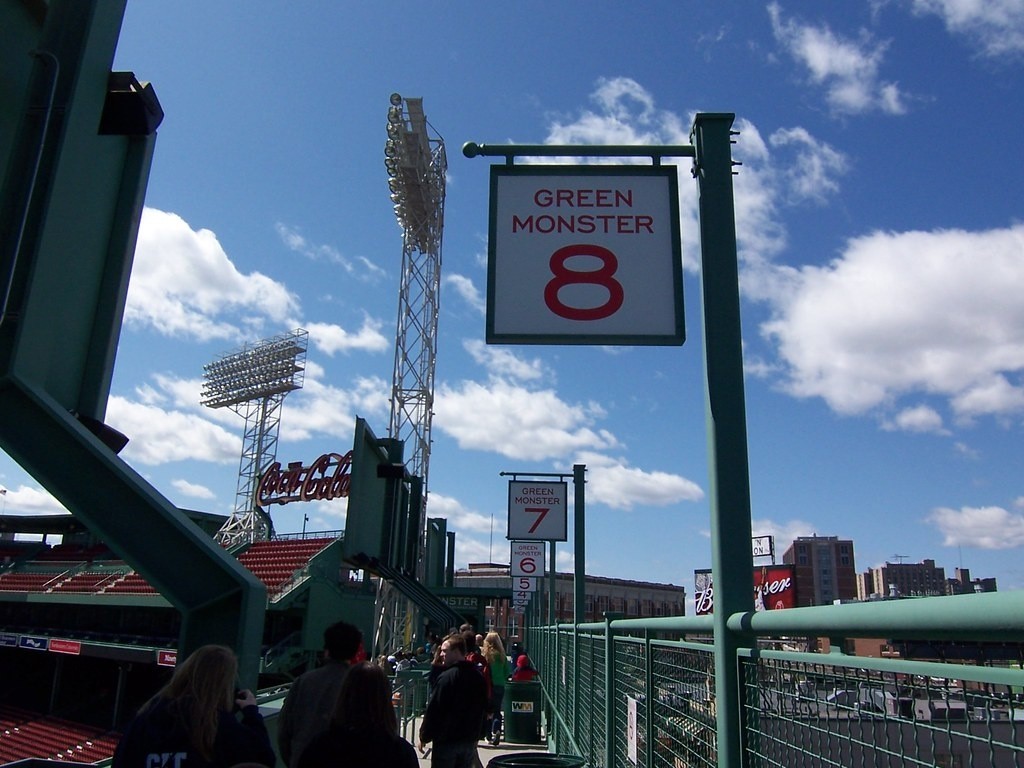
[471,748,483,768]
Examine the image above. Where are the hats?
[388,656,397,663]
[322,619,365,653]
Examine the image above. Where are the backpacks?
[465,644,493,699]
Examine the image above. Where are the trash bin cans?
[498,677,546,746]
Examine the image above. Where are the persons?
[293,660,420,768]
[418,635,495,768]
[278,618,366,768]
[111,644,278,768]
[376,621,540,748]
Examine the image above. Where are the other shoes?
[493,731,501,745]
